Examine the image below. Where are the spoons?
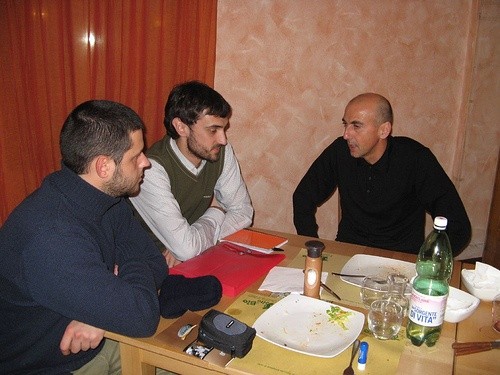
[332,273,388,284]
[342,340,360,375]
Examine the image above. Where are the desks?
[104,227,500,375]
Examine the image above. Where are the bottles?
[303,241,325,300]
[383,275,409,321]
[406,216,453,346]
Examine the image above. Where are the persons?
[126,80,255,263]
[0,99,169,375]
[293,94,473,260]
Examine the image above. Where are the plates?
[341,253,418,295]
[251,293,366,357]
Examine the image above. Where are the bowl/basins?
[461,261,500,300]
[444,285,480,323]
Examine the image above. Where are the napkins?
[258,266,328,296]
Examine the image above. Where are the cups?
[492,294,500,333]
[368,300,402,339]
[360,276,388,310]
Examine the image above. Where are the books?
[220,228,288,254]
